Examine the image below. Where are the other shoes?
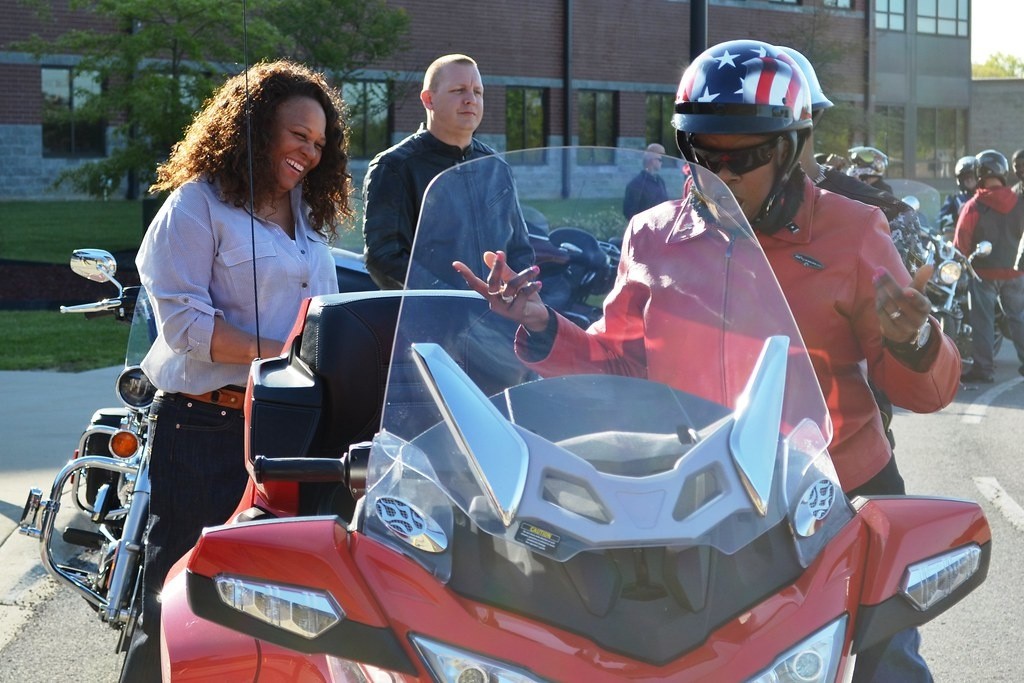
[1019,365,1024,375]
[960,370,994,384]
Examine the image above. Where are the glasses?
[656,158,662,161]
[690,135,787,174]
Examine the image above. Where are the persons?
[623,142,1024,383]
[118,56,352,683]
[451,38,962,496]
[773,44,937,683]
[363,53,538,442]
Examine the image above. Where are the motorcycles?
[22,248,156,656]
[160,146,1003,683]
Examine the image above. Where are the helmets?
[955,155,975,190]
[776,46,834,111]
[846,146,888,179]
[671,40,813,196]
[976,149,1009,184]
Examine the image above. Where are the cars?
[331,248,374,296]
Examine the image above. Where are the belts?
[181,388,245,410]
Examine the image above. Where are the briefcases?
[75,407,134,513]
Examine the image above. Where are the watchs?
[886,317,932,352]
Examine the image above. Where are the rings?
[488,289,500,295]
[888,307,903,321]
[501,291,517,303]
